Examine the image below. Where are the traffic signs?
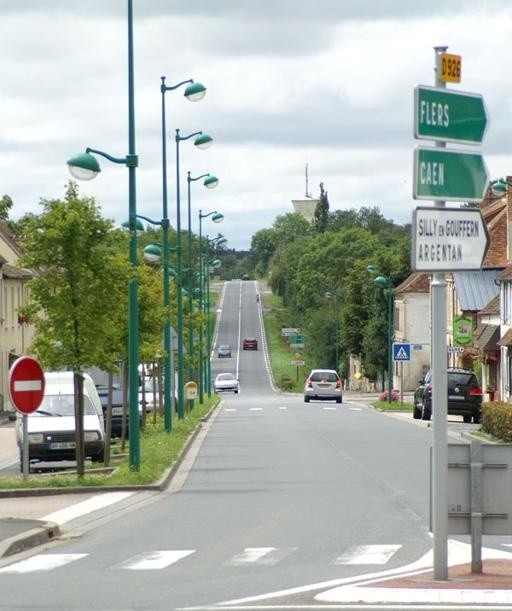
[280,327,305,348]
[290,360,305,366]
[413,84,491,147]
[412,145,490,203]
[411,207,490,274]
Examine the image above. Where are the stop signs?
[7,357,45,416]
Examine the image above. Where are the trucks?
[16,372,106,474]
[138,363,178,413]
[81,360,143,440]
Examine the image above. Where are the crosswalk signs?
[391,341,412,360]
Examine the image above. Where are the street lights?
[159,171,228,412]
[141,129,213,421]
[121,75,207,433]
[367,266,391,406]
[324,292,343,375]
[67,0,140,474]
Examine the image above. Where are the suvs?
[242,338,258,351]
[413,368,483,424]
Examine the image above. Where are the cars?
[212,373,240,395]
[242,275,249,281]
[303,369,345,404]
[217,345,232,358]
